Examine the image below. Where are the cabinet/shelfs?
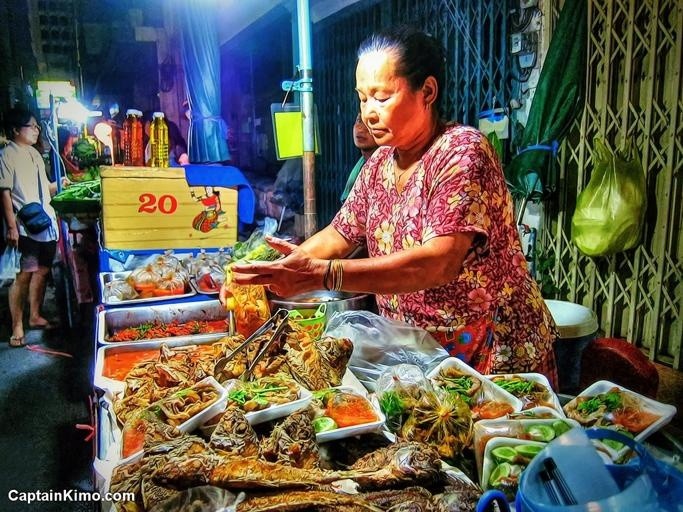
[59,214,95,327]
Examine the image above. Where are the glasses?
[22,123,42,131]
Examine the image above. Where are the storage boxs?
[98,164,238,251]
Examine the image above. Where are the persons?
[0,105,57,348]
[165,120,189,168]
[143,119,154,164]
[49,128,80,181]
[229,24,562,394]
[189,186,227,233]
[340,103,382,204]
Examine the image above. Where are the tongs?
[212,307,291,382]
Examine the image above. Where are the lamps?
[60,99,89,123]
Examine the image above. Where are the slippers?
[31,320,62,330]
[9,335,26,348]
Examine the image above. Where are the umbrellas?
[176,0,233,164]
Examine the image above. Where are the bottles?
[149,112,169,168]
[135,111,144,166]
[124,109,139,166]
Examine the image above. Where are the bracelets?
[322,257,346,293]
